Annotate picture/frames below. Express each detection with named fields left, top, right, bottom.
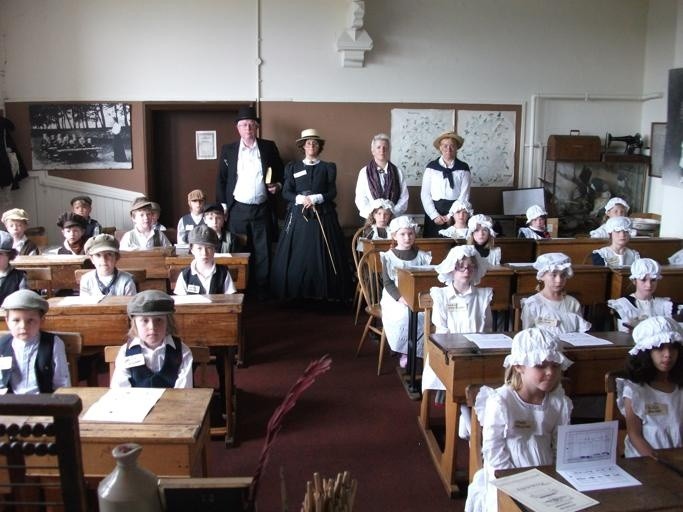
left=648, top=122, right=666, bottom=178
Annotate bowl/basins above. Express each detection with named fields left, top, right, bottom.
left=629, top=217, right=660, bottom=231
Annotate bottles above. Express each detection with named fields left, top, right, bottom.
left=95, top=441, right=164, bottom=512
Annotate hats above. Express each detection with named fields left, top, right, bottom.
left=368, top=198, right=501, bottom=285
left=0, top=207, right=30, bottom=251
left=125, top=289, right=176, bottom=318
left=56, top=195, right=120, bottom=257
left=129, top=190, right=223, bottom=247
left=525, top=205, right=547, bottom=223
left=629, top=317, right=681, bottom=356
left=503, top=326, right=573, bottom=371
left=2, top=289, right=50, bottom=312
left=603, top=197, right=630, bottom=214
left=295, top=128, right=325, bottom=146
left=234, top=108, right=261, bottom=124
left=603, top=214, right=631, bottom=233
left=629, top=258, right=662, bottom=281
left=534, top=253, right=574, bottom=281
left=434, top=132, right=464, bottom=152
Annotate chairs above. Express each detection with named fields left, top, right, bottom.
left=351, top=226, right=367, bottom=327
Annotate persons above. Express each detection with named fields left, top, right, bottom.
left=465, top=214, right=502, bottom=267
left=592, top=216, right=641, bottom=267
left=40, top=132, right=98, bottom=163
left=365, top=199, right=396, bottom=302
left=616, top=316, right=681, bottom=458
left=438, top=199, right=474, bottom=239
left=177, top=192, right=237, bottom=253
left=79, top=233, right=138, bottom=296
left=216, top=106, right=285, bottom=302
left=421, top=245, right=496, bottom=462
left=667, top=249, right=681, bottom=265
left=2, top=231, right=29, bottom=305
left=518, top=205, right=552, bottom=240
left=380, top=216, right=436, bottom=369
left=110, top=290, right=193, bottom=389
left=2, top=289, right=71, bottom=394
left=70, top=196, right=102, bottom=245
left=174, top=224, right=236, bottom=419
left=46, top=212, right=95, bottom=297
left=355, top=132, right=409, bottom=222
left=111, top=116, right=128, bottom=161
left=420, top=130, right=472, bottom=239
left=119, top=197, right=172, bottom=252
left=270, top=129, right=352, bottom=304
left=519, top=253, right=591, bottom=337
left=465, top=328, right=573, bottom=509
left=608, top=258, right=681, bottom=333
left=2, top=208, right=45, bottom=296
left=589, top=199, right=637, bottom=238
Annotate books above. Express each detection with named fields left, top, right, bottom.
left=265, top=166, right=273, bottom=184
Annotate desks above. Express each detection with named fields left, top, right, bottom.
left=41, top=143, right=104, bottom=165
left=359, top=213, right=683, bottom=512
left=0, top=230, right=250, bottom=479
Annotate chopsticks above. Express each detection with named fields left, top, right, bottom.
left=299, top=470, right=358, bottom=511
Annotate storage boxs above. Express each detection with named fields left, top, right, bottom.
left=547, top=133, right=602, bottom=161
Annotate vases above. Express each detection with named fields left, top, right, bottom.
left=97, top=443, right=162, bottom=511
